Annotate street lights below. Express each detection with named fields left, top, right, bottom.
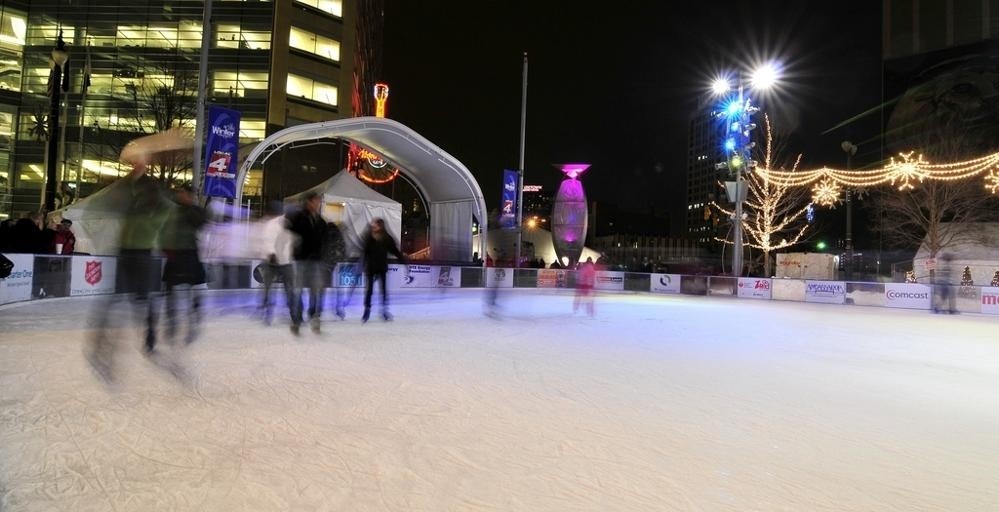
left=714, top=69, right=776, bottom=295
left=841, top=141, right=857, bottom=294
left=32, top=27, right=69, bottom=296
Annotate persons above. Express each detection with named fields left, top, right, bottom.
left=594, top=251, right=609, bottom=270
left=48, top=218, right=76, bottom=255
left=571, top=256, right=596, bottom=319
left=4, top=211, right=48, bottom=253
left=151, top=181, right=207, bottom=347
left=582, top=257, right=594, bottom=270
left=484, top=251, right=492, bottom=267
left=929, top=253, right=963, bottom=316
left=550, top=259, right=561, bottom=269
left=307, top=220, right=348, bottom=319
left=628, top=256, right=669, bottom=273
left=258, top=204, right=307, bottom=324
left=472, top=251, right=484, bottom=266
left=357, top=217, right=406, bottom=322
left=495, top=255, right=503, bottom=267
left=83, top=131, right=173, bottom=375
left=538, top=257, right=546, bottom=268
left=280, top=189, right=332, bottom=334
